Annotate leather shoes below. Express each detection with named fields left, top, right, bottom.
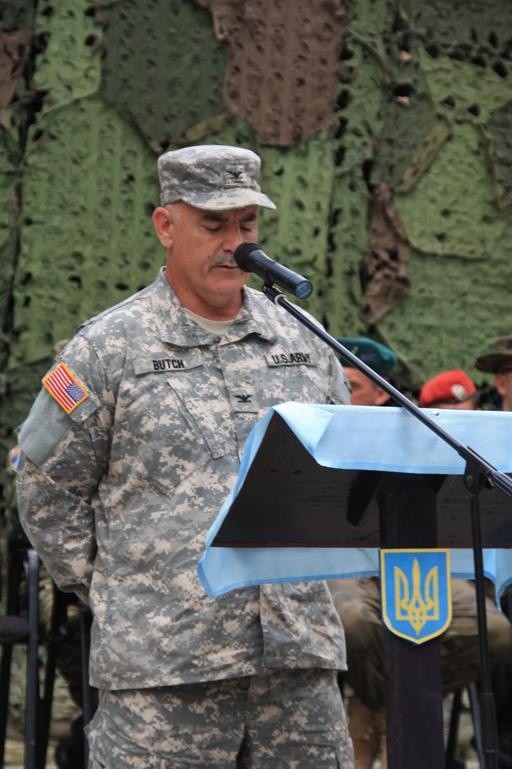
left=53, top=715, right=89, bottom=768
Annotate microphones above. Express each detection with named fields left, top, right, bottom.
left=233, top=241, right=313, bottom=299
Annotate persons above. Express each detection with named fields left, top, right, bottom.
left=17, top=141, right=357, bottom=769
left=1, top=332, right=512, bottom=769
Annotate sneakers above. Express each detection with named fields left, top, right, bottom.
left=346, top=698, right=389, bottom=768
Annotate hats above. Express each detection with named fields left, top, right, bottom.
left=332, top=335, right=398, bottom=376
left=158, top=145, right=277, bottom=213
left=475, top=336, right=512, bottom=374
left=418, top=368, right=477, bottom=408
